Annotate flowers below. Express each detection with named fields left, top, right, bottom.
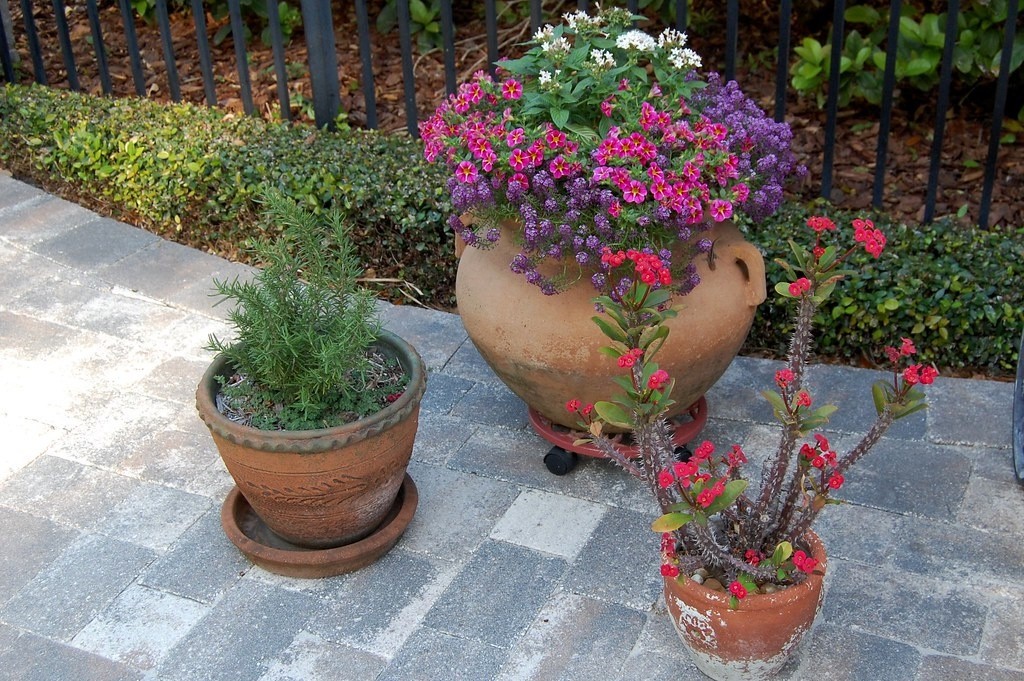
left=567, top=216, right=939, bottom=610
left=416, top=2, right=807, bottom=321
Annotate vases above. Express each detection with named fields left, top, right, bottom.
left=660, top=513, right=827, bottom=681
left=454, top=208, right=767, bottom=437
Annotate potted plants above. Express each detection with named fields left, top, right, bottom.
left=196, top=182, right=427, bottom=548
